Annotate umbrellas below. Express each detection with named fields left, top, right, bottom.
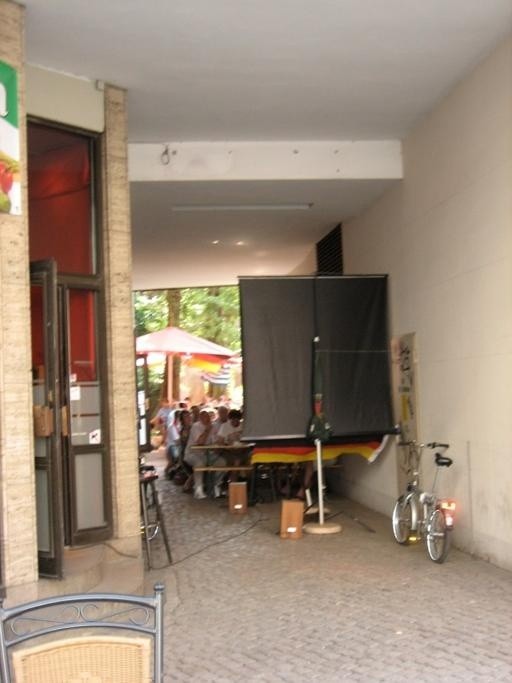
left=137, top=324, right=234, bottom=404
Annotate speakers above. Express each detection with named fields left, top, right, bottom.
left=281, top=499, right=305, bottom=540
left=229, top=482, right=247, bottom=514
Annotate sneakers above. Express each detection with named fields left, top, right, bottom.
left=193, top=480, right=223, bottom=499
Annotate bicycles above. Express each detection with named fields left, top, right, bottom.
left=392, top=440, right=456, bottom=563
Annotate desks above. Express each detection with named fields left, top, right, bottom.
left=191, top=441, right=344, bottom=502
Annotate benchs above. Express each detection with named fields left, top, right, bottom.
left=192, top=465, right=345, bottom=505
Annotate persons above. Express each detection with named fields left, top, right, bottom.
left=279, top=460, right=315, bottom=501
left=148, top=395, right=249, bottom=500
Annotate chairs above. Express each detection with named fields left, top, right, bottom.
left=0, top=583, right=165, bottom=683
